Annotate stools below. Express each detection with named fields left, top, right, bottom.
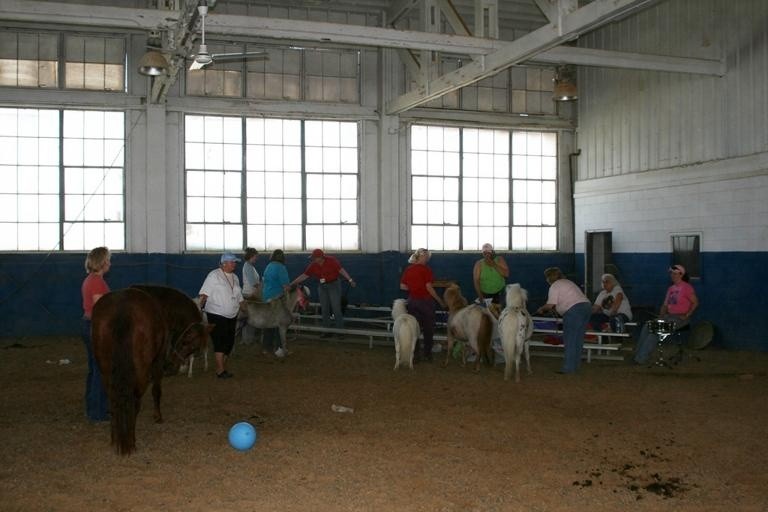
left=668, top=325, right=699, bottom=366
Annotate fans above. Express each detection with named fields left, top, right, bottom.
left=151, top=5, right=274, bottom=73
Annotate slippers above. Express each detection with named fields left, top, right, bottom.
left=217, top=369, right=234, bottom=378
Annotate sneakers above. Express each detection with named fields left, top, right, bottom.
left=319, top=332, right=333, bottom=338
left=336, top=333, right=345, bottom=339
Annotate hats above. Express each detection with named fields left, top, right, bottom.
left=220, top=251, right=242, bottom=265
left=668, top=264, right=686, bottom=275
left=481, top=243, right=495, bottom=254
left=310, top=248, right=324, bottom=256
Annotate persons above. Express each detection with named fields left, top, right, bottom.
left=400, top=248, right=445, bottom=363
left=80, top=246, right=113, bottom=421
left=198, top=253, right=247, bottom=378
left=289, top=249, right=356, bottom=340
left=261, top=249, right=291, bottom=353
left=633, top=264, right=699, bottom=366
left=590, top=274, right=633, bottom=344
left=537, top=267, right=593, bottom=374
left=241, top=248, right=260, bottom=300
left=473, top=243, right=510, bottom=305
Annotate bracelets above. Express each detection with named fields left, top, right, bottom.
left=349, top=278, right=353, bottom=283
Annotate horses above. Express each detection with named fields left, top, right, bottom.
left=182, top=295, right=210, bottom=379
left=442, top=283, right=494, bottom=373
left=498, top=283, right=534, bottom=382
left=89, top=284, right=217, bottom=460
left=236, top=282, right=312, bottom=356
left=389, top=297, right=421, bottom=376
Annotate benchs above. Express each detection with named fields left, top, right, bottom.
left=290, top=299, right=640, bottom=368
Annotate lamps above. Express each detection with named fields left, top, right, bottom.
left=139, top=23, right=173, bottom=79
left=549, top=63, right=582, bottom=105
